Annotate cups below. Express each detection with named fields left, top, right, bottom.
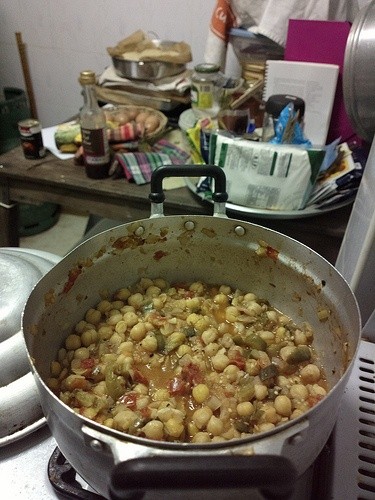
left=217, top=105, right=251, bottom=137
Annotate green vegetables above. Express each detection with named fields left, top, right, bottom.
left=72, top=299, right=313, bottom=434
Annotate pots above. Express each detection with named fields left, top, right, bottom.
left=21, top=164, right=363, bottom=500
left=113, top=56, right=186, bottom=80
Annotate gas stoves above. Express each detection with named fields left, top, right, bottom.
left=48, top=340, right=375, bottom=500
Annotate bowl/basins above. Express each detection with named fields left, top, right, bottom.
left=184, top=144, right=355, bottom=218
left=221, top=74, right=245, bottom=97
left=104, top=105, right=169, bottom=145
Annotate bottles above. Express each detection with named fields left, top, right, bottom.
left=262, top=95, right=305, bottom=143
left=80, top=71, right=115, bottom=180
left=190, top=63, right=225, bottom=120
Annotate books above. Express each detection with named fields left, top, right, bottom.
left=285, top=19, right=362, bottom=149
left=263, top=60, right=339, bottom=150
left=307, top=137, right=364, bottom=209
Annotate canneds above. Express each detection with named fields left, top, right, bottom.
left=18, top=120, right=45, bottom=159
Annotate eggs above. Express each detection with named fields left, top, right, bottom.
left=103, top=107, right=160, bottom=133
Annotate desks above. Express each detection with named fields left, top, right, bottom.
left=0, top=111, right=214, bottom=249
